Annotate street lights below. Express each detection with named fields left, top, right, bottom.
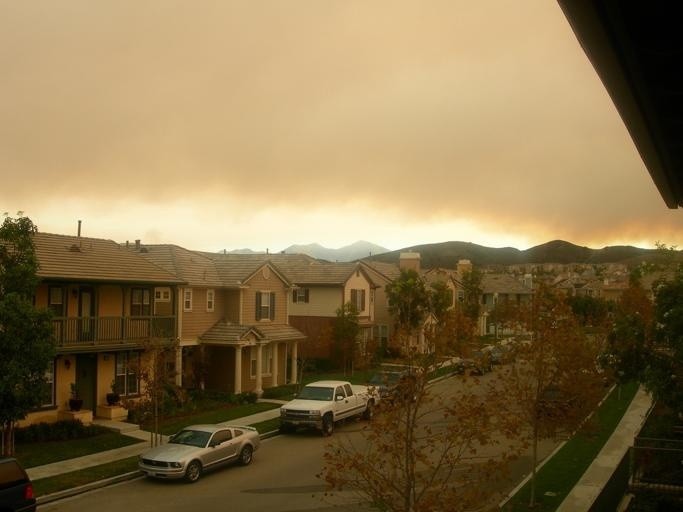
left=153, top=337, right=184, bottom=446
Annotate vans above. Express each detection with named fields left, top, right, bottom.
left=1, top=457, right=36, bottom=512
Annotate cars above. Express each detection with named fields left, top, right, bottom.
left=136, top=423, right=260, bottom=481
left=454, top=337, right=535, bottom=376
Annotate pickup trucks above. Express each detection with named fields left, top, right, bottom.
left=280, top=380, right=379, bottom=437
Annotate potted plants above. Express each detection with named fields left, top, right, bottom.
left=106, top=380, right=124, bottom=403
left=68, top=383, right=82, bottom=411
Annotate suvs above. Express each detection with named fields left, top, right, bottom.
left=369, top=370, right=417, bottom=406
